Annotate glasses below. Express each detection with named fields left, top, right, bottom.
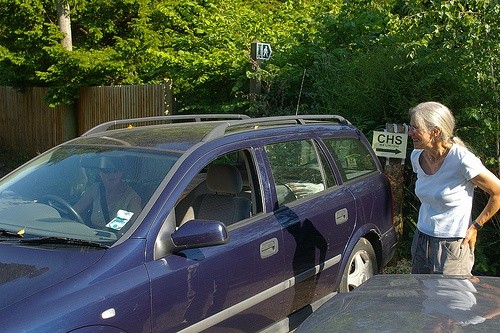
left=408, top=125, right=423, bottom=134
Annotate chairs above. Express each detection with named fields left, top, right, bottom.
left=188, top=163, right=251, bottom=226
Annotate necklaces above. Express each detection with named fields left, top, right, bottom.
left=428, top=146, right=448, bottom=165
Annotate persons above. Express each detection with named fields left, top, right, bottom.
left=69, top=168, right=141, bottom=230
left=416, top=275, right=500, bottom=333
left=410, top=102, right=500, bottom=275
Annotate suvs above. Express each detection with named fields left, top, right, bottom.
left=0, top=115, right=397, bottom=333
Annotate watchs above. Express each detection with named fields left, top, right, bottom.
left=473, top=220, right=484, bottom=231
